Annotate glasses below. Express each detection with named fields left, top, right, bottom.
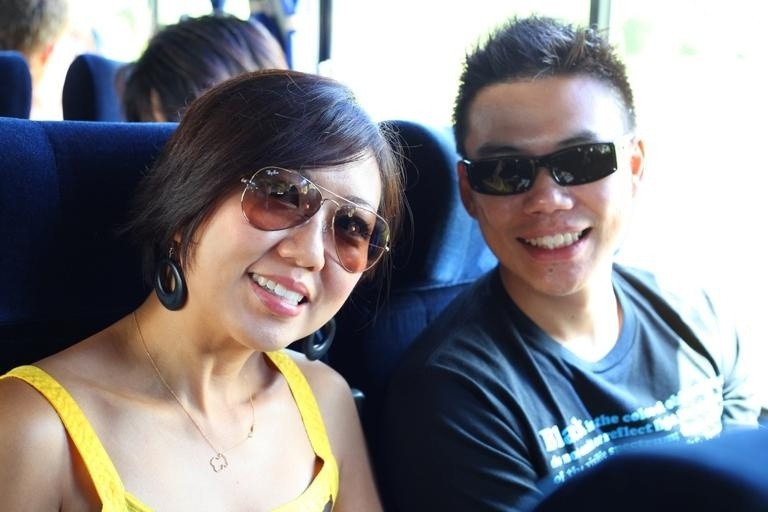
left=460, top=130, right=635, bottom=198
left=239, top=166, right=393, bottom=274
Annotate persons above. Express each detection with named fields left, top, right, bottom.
left=0, top=0, right=74, bottom=124
left=115, top=10, right=289, bottom=121
left=385, top=15, right=763, bottom=509
left=2, top=66, right=409, bottom=511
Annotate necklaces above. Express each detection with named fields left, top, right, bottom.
left=132, top=309, right=259, bottom=473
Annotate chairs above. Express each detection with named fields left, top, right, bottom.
left=0, top=53, right=768, bottom=512
